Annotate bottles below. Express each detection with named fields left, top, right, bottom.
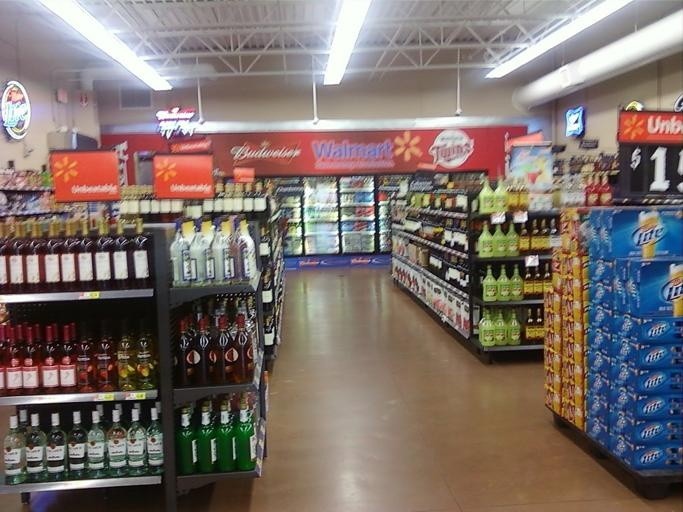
left=477, top=170, right=612, bottom=348
left=0, top=179, right=284, bottom=486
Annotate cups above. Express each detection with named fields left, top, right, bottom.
left=668, top=265, right=682, bottom=317
left=637, top=212, right=657, bottom=259
